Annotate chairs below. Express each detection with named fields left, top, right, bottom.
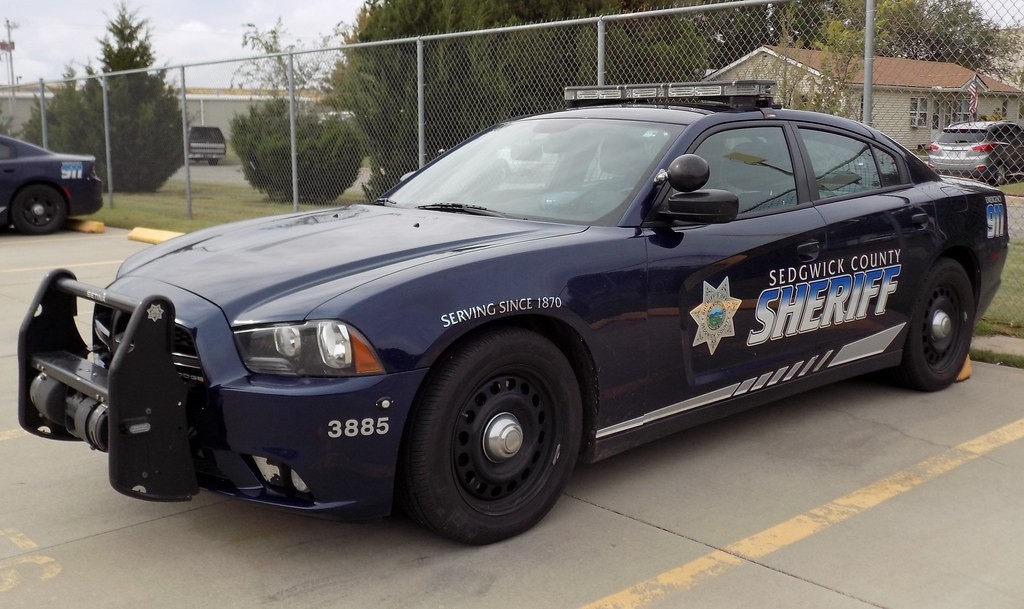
left=725, top=142, right=778, bottom=209
left=598, top=131, right=644, bottom=182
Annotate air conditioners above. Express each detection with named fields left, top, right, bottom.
left=910, top=116, right=925, bottom=128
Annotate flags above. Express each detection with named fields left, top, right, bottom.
left=962, top=75, right=979, bottom=113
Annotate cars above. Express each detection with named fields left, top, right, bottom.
left=187, top=125, right=229, bottom=166
left=927, top=118, right=1024, bottom=186
left=13, top=80, right=1010, bottom=546
left=0, top=135, right=105, bottom=235
